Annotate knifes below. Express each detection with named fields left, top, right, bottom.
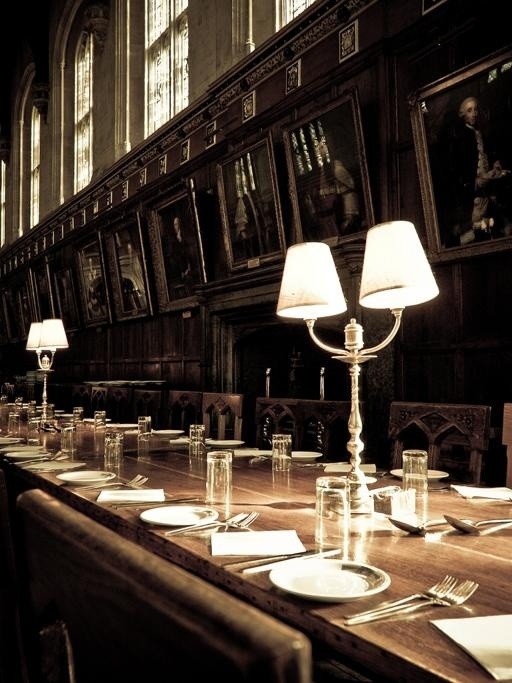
left=112, top=496, right=199, bottom=509
left=223, top=547, right=342, bottom=575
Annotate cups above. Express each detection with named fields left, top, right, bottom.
left=313, top=475, right=350, bottom=544
left=272, top=433, right=293, bottom=471
left=0, top=394, right=206, bottom=465
left=207, top=449, right=234, bottom=505
left=400, top=447, right=428, bottom=499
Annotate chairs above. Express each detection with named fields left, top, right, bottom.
left=0, top=386, right=512, bottom=678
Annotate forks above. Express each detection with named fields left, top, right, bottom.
left=343, top=578, right=481, bottom=630
left=82, top=473, right=149, bottom=489
left=342, top=573, right=460, bottom=619
left=165, top=509, right=261, bottom=536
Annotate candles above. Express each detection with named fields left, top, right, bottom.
left=263, top=367, right=271, bottom=397
left=318, top=366, right=327, bottom=400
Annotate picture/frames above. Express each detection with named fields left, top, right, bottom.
left=1, top=209, right=155, bottom=347
left=281, top=86, right=375, bottom=250
left=213, top=130, right=288, bottom=273
left=141, top=176, right=210, bottom=315
left=407, top=43, right=509, bottom=263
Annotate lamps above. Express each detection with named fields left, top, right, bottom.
left=25, top=319, right=72, bottom=423
left=276, top=218, right=441, bottom=517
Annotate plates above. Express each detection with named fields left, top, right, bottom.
left=207, top=439, right=244, bottom=448
left=257, top=450, right=323, bottom=461
left=391, top=467, right=449, bottom=483
left=56, top=469, right=116, bottom=485
left=272, top=559, right=388, bottom=599
left=141, top=504, right=220, bottom=525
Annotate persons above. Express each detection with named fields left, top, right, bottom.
left=447, top=94, right=501, bottom=240
left=167, top=215, right=198, bottom=282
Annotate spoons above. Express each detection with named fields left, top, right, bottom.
left=442, top=514, right=512, bottom=533
left=387, top=513, right=470, bottom=534
